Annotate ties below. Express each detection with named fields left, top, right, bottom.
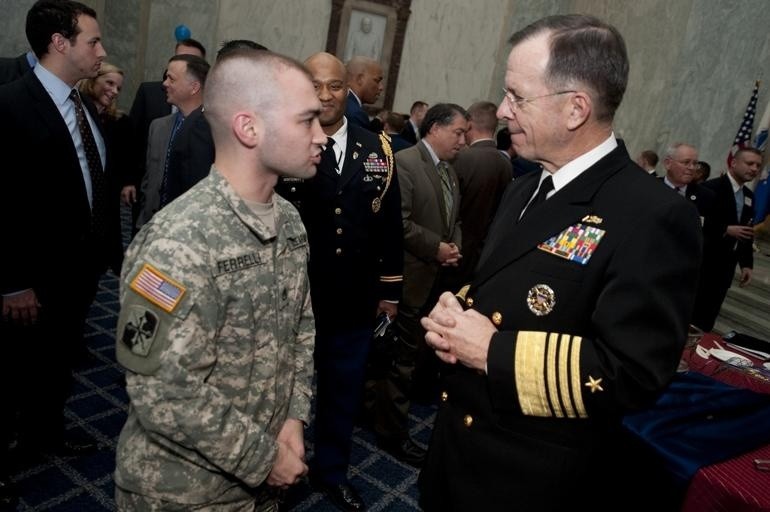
left=439, top=162, right=459, bottom=232
left=519, top=175, right=554, bottom=221
left=323, top=136, right=340, bottom=171
left=69, top=89, right=107, bottom=210
left=160, top=118, right=185, bottom=208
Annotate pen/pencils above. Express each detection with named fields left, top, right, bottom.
left=733, top=217, right=753, bottom=251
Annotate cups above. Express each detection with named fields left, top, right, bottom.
left=675, top=324, right=704, bottom=373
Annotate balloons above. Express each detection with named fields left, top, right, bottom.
left=173, top=25, right=192, bottom=43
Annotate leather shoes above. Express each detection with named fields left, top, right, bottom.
left=376, top=436, right=427, bottom=468
left=1, top=480, right=21, bottom=510
left=35, top=434, right=102, bottom=456
left=309, top=471, right=367, bottom=510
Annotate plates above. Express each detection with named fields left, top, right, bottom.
left=711, top=348, right=754, bottom=370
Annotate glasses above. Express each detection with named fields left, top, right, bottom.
left=502, top=84, right=578, bottom=111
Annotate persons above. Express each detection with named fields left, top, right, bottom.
left=0, top=45, right=39, bottom=85
left=1, top=2, right=122, bottom=474
left=77, top=11, right=768, bottom=510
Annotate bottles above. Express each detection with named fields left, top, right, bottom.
left=742, top=217, right=755, bottom=240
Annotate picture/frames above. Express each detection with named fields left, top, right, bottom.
left=325, top=0, right=412, bottom=116
